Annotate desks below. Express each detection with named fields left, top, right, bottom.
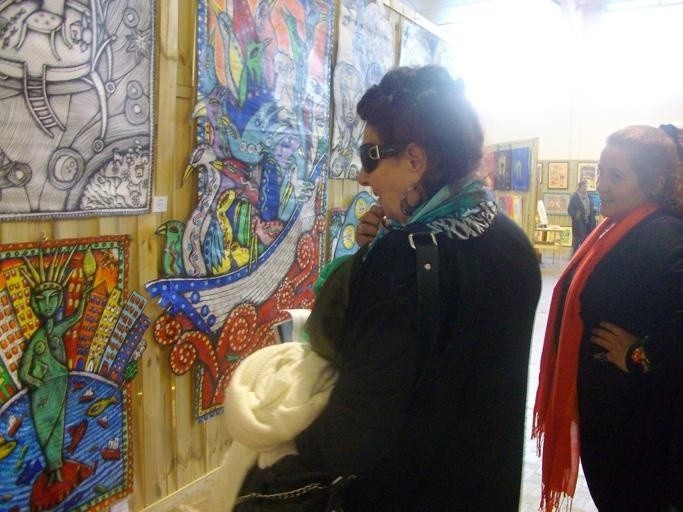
left=535, top=224, right=565, bottom=267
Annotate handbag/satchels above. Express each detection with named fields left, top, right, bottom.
left=232, top=455, right=331, bottom=511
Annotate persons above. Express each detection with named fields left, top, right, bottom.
left=529, top=124, right=682, bottom=512
left=567, top=180, right=597, bottom=253
left=201, top=66, right=540, bottom=512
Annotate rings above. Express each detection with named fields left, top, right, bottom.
left=599, top=352, right=607, bottom=363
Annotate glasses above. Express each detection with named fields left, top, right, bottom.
left=358, top=138, right=405, bottom=174
